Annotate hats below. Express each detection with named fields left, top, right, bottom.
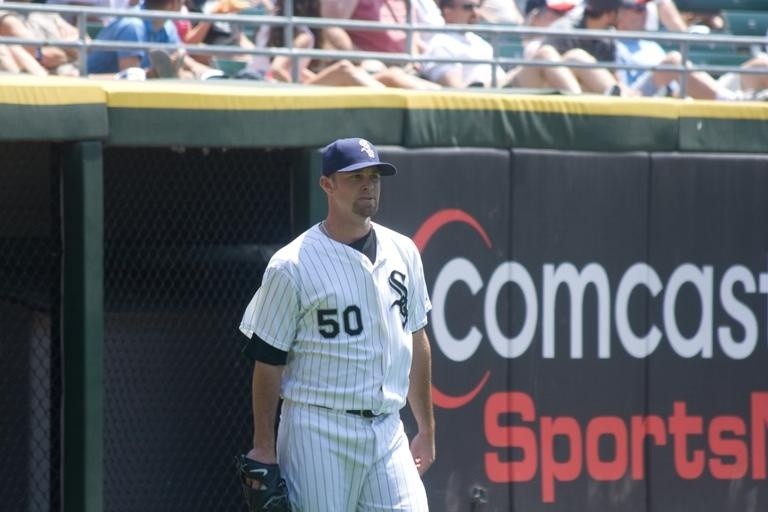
left=317, top=134, right=400, bottom=180
left=543, top=0, right=578, bottom=13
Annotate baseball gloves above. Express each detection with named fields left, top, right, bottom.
left=236, top=453, right=292, bottom=512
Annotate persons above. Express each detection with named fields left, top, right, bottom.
left=235, top=138, right=437, bottom=512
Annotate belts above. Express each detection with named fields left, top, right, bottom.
left=303, top=399, right=386, bottom=420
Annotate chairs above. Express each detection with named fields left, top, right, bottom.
left=79, top=0, right=767, bottom=105
